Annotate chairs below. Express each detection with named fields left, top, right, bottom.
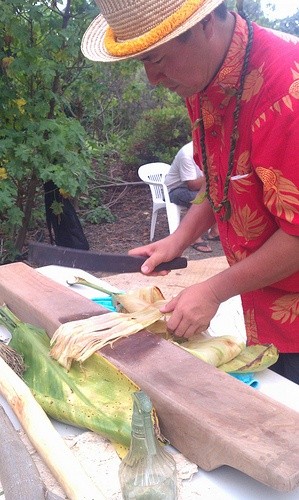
left=138, top=162, right=187, bottom=241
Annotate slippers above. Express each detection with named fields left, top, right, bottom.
left=190, top=241, right=213, bottom=253
left=208, top=234, right=221, bottom=241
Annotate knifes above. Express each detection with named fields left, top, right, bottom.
left=27, top=241, right=188, bottom=274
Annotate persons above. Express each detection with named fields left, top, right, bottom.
left=81, top=0, right=299, bottom=386
left=161, top=142, right=220, bottom=252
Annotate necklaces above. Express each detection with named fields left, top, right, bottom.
left=199, top=9, right=253, bottom=221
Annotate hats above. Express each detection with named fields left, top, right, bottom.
left=79, top=0, right=231, bottom=63
